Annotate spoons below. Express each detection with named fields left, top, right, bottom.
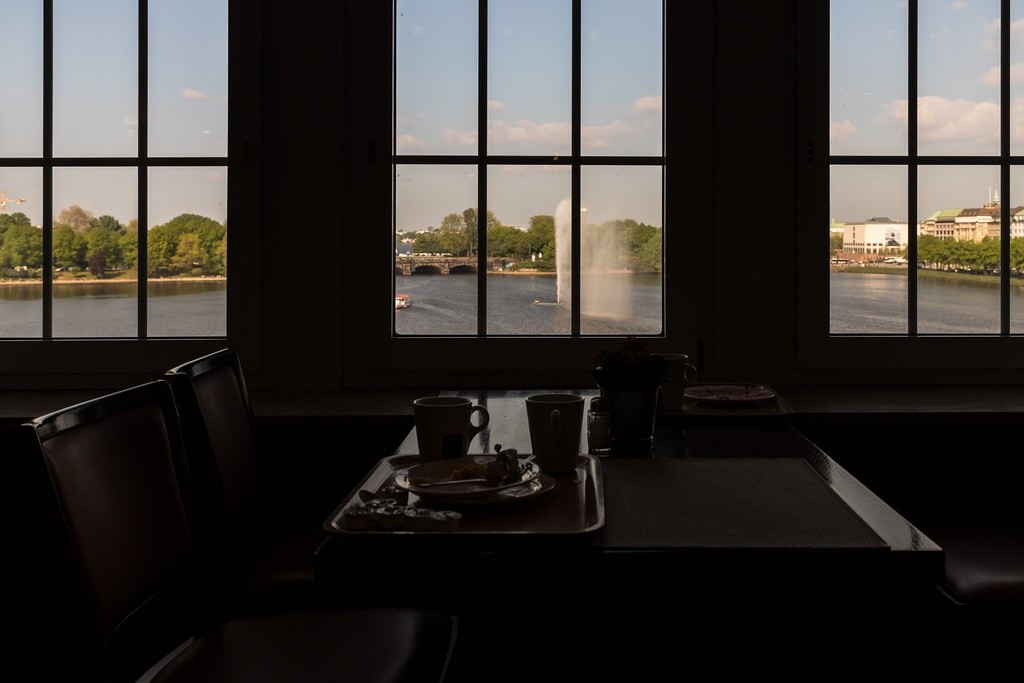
left=416, top=478, right=487, bottom=488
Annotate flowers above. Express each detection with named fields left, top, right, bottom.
left=591, top=339, right=655, bottom=371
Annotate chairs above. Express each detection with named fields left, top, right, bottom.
left=925, top=514, right=1023, bottom=683
left=15, top=379, right=468, bottom=682
left=164, top=348, right=508, bottom=628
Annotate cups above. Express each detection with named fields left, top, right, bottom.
left=526, top=392, right=585, bottom=472
left=650, top=353, right=696, bottom=412
left=412, top=395, right=489, bottom=463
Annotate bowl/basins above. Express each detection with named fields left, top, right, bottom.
left=343, top=486, right=462, bottom=532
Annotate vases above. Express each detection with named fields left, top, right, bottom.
left=590, top=371, right=672, bottom=460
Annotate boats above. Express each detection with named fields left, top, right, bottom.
left=394, top=293, right=410, bottom=310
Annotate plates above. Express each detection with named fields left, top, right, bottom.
left=457, top=473, right=557, bottom=504
left=684, top=385, right=775, bottom=408
left=394, top=456, right=540, bottom=498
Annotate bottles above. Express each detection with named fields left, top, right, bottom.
left=588, top=397, right=613, bottom=458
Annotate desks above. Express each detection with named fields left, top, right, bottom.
left=310, top=387, right=947, bottom=683
